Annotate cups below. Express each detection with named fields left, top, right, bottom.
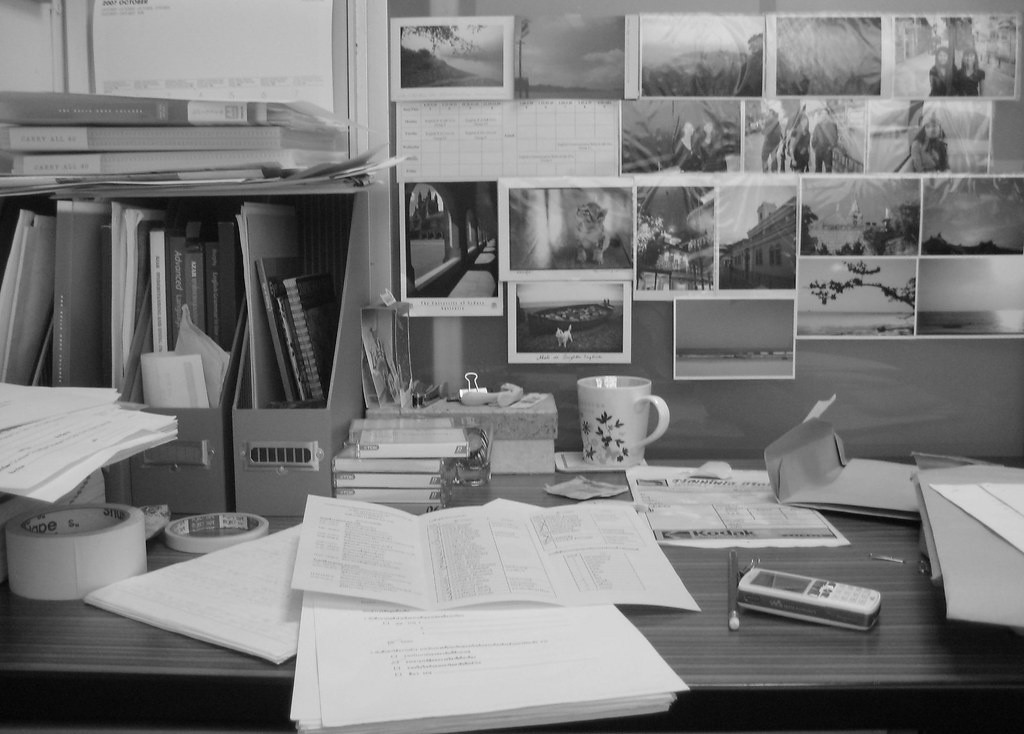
left=575, top=375, right=671, bottom=468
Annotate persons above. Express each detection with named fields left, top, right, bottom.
left=677, top=34, right=984, bottom=170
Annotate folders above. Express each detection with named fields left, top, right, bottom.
left=0, top=87, right=355, bottom=175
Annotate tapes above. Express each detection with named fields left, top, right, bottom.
left=5, top=502, right=148, bottom=601
left=164, top=512, right=270, bottom=553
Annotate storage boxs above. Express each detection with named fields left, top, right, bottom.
left=365, top=391, right=557, bottom=474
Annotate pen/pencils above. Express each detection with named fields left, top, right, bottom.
left=727, top=547, right=740, bottom=631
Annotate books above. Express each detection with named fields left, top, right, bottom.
left=0, top=200, right=1024, bottom=734
left=1, top=91, right=412, bottom=196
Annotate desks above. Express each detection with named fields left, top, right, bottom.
left=0, top=460, right=1024, bottom=689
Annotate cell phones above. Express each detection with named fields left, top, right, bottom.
left=737, top=567, right=882, bottom=631
